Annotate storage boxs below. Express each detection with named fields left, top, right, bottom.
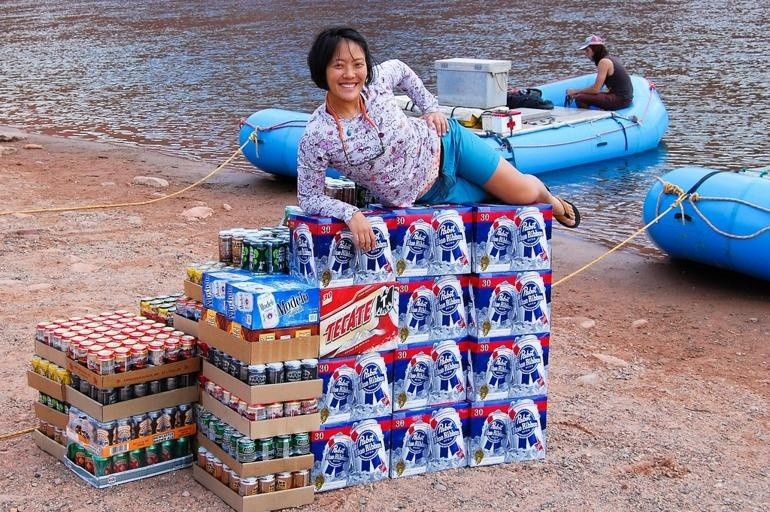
left=435, top=58, right=512, bottom=109
left=202, top=202, right=553, bottom=493
left=481, top=111, right=521, bottom=134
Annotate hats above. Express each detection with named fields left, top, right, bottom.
left=579, top=35, right=604, bottom=50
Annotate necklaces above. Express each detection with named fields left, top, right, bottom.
left=328, top=102, right=361, bottom=137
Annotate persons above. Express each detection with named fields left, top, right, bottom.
left=563, top=33, right=635, bottom=111
left=295, top=26, right=581, bottom=253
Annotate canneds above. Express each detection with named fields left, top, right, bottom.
left=196, top=446, right=309, bottom=496
left=217, top=226, right=291, bottom=275
left=483, top=207, right=553, bottom=271
left=176, top=295, right=202, bottom=322
left=207, top=345, right=318, bottom=386
left=484, top=271, right=550, bottom=327
left=325, top=353, right=388, bottom=413
left=210, top=280, right=227, bottom=299
left=323, top=173, right=375, bottom=210
left=479, top=398, right=544, bottom=454
left=403, top=340, right=463, bottom=401
left=70, top=372, right=194, bottom=404
left=193, top=401, right=309, bottom=463
left=204, top=381, right=318, bottom=421
left=486, top=333, right=545, bottom=391
left=327, top=216, right=395, bottom=281
left=35, top=310, right=195, bottom=375
left=195, top=338, right=209, bottom=390
left=68, top=437, right=194, bottom=477
left=399, top=210, right=472, bottom=275
left=38, top=391, right=70, bottom=416
left=68, top=403, right=193, bottom=447
left=402, top=407, right=466, bottom=463
left=140, top=293, right=186, bottom=327
left=32, top=354, right=67, bottom=386
left=186, top=260, right=234, bottom=286
left=234, top=292, right=254, bottom=312
left=257, top=292, right=279, bottom=329
left=291, top=223, right=316, bottom=280
left=320, top=418, right=387, bottom=480
left=405, top=275, right=467, bottom=334
left=39, top=420, right=68, bottom=447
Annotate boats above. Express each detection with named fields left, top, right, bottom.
left=643, top=165, right=769, bottom=281
left=239, top=66, right=670, bottom=184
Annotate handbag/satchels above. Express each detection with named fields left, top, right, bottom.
left=507, top=89, right=541, bottom=109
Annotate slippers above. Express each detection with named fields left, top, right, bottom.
left=553, top=196, right=580, bottom=229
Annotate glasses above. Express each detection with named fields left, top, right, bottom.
left=340, top=116, right=385, bottom=167
